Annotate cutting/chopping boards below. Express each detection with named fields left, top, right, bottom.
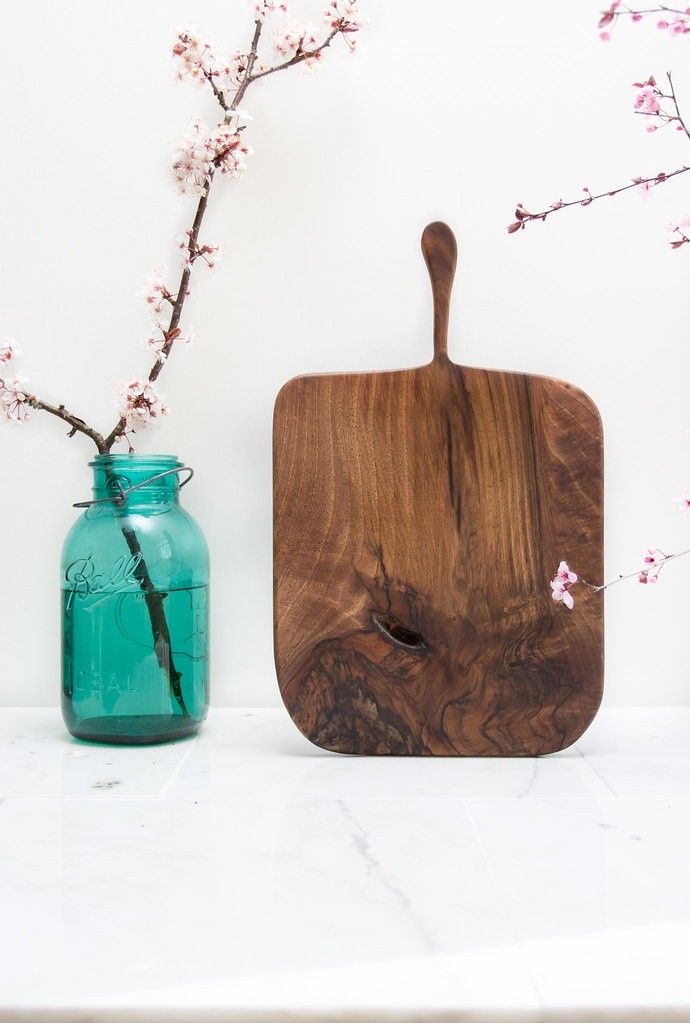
left=273, top=220, right=606, bottom=757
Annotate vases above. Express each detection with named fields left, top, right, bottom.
left=59, top=455, right=209, bottom=739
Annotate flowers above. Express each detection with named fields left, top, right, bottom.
left=505, top=1, right=690, bottom=264
left=0, top=2, right=358, bottom=726
left=550, top=496, right=690, bottom=610
